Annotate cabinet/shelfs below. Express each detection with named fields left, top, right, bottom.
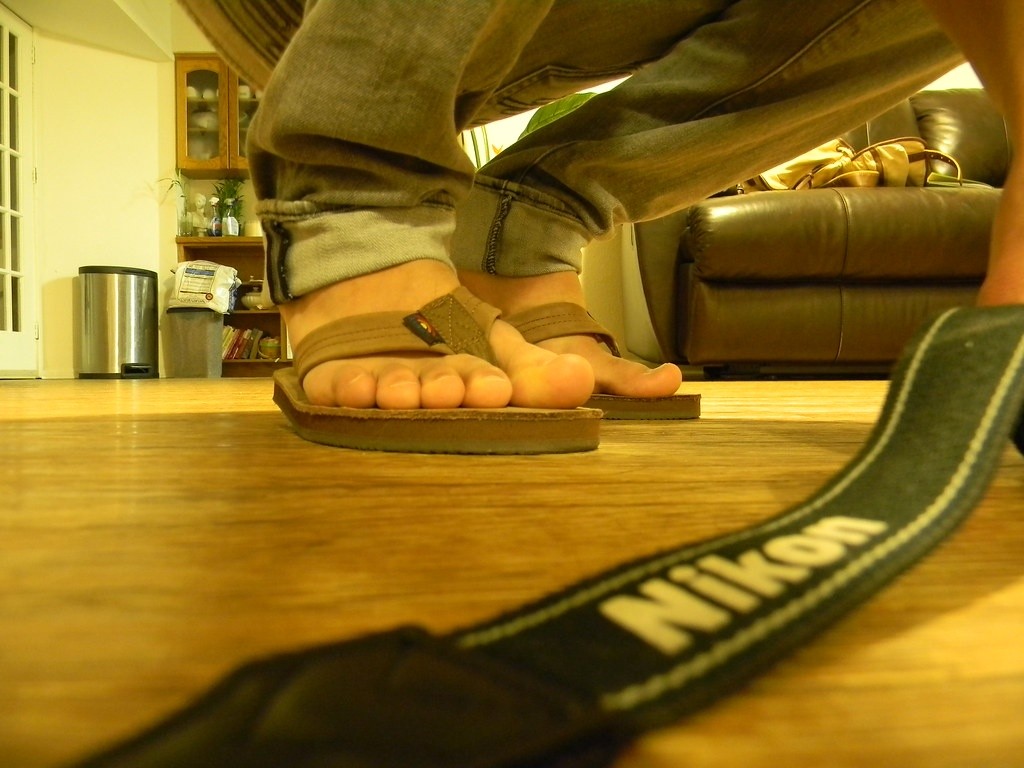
left=174, top=236, right=294, bottom=378
left=174, top=51, right=264, bottom=181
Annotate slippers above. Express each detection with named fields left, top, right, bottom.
left=271, top=281, right=605, bottom=456
left=497, top=301, right=702, bottom=421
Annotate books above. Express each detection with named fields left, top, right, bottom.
left=222, top=325, right=262, bottom=359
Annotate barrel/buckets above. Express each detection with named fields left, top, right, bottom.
left=258, top=343, right=281, bottom=359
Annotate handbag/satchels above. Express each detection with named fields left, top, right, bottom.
left=725, top=136, right=964, bottom=195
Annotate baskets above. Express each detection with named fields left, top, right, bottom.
left=259, top=343, right=282, bottom=359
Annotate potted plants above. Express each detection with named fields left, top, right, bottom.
left=157, top=167, right=195, bottom=236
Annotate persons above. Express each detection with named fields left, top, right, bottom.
left=187, top=193, right=208, bottom=230
left=181, top=0, right=1024, bottom=454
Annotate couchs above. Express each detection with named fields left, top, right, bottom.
left=619, top=89, right=1013, bottom=382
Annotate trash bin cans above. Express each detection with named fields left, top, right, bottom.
left=165, top=306, right=225, bottom=378
left=77, top=264, right=162, bottom=379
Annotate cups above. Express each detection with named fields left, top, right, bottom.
left=239, top=86, right=250, bottom=98
